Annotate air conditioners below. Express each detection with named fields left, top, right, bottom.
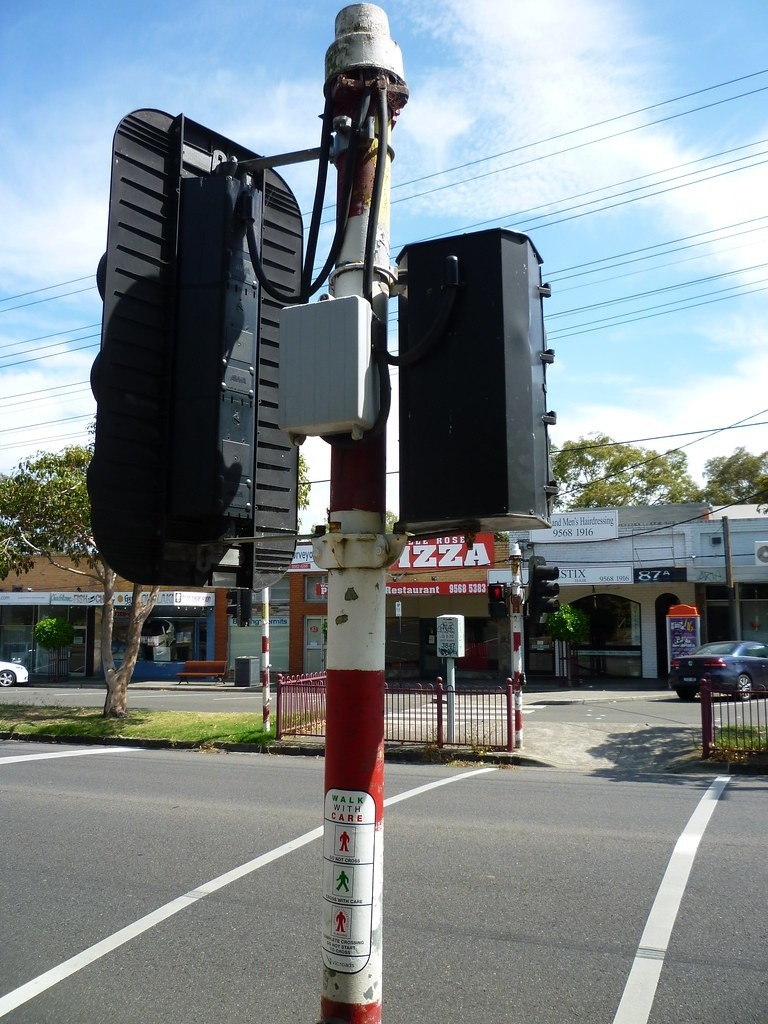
left=755, top=541, right=768, bottom=566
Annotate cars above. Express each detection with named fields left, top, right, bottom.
left=669, top=641, right=768, bottom=702
left=5, top=642, right=35, bottom=670
left=0, top=661, right=29, bottom=686
left=139, top=619, right=176, bottom=647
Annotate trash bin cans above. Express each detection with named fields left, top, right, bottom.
left=235, top=656, right=261, bottom=687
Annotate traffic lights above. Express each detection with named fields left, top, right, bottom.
left=487, top=581, right=508, bottom=618
left=529, top=557, right=560, bottom=623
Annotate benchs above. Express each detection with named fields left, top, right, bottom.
left=176, top=661, right=229, bottom=685
